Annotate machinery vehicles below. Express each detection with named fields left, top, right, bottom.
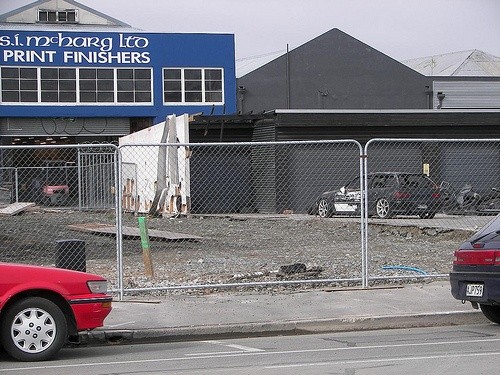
left=37, top=160, right=70, bottom=205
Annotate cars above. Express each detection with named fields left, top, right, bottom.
left=449, top=213, right=500, bottom=324
left=316, top=169, right=444, bottom=218
left=0, top=262, right=114, bottom=361
